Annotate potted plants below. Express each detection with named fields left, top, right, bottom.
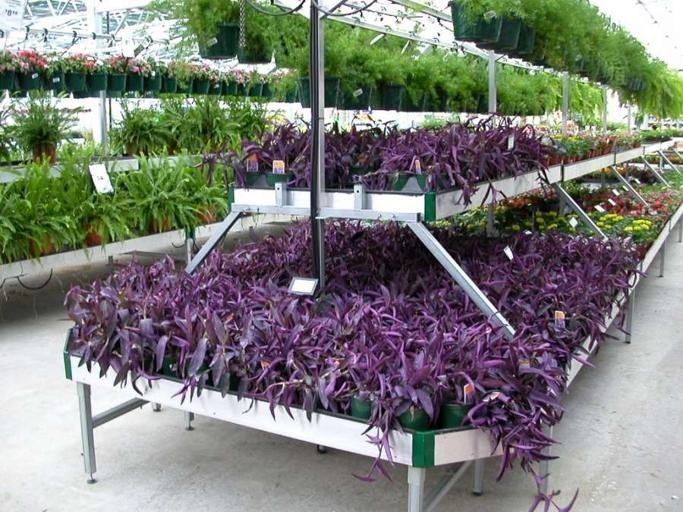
left=0, top=0, right=683, bottom=512
